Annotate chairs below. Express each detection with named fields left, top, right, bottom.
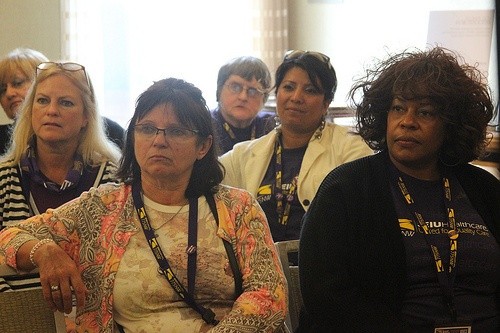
left=0, top=239, right=301, bottom=333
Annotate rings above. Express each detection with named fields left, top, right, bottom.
left=50, top=285, right=59, bottom=291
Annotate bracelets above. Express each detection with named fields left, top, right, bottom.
left=29, top=239, right=57, bottom=267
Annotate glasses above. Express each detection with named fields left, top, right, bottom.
left=132, top=124, right=198, bottom=139
left=284, top=50, right=336, bottom=72
left=35, top=61, right=91, bottom=89
left=224, top=82, right=264, bottom=97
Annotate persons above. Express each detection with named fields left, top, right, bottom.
left=218, top=49, right=382, bottom=267
left=0, top=78, right=291, bottom=333
left=0, top=48, right=126, bottom=232
left=209, top=57, right=281, bottom=157
left=298, top=46, right=500, bottom=333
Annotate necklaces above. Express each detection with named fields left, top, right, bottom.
left=141, top=189, right=188, bottom=231
